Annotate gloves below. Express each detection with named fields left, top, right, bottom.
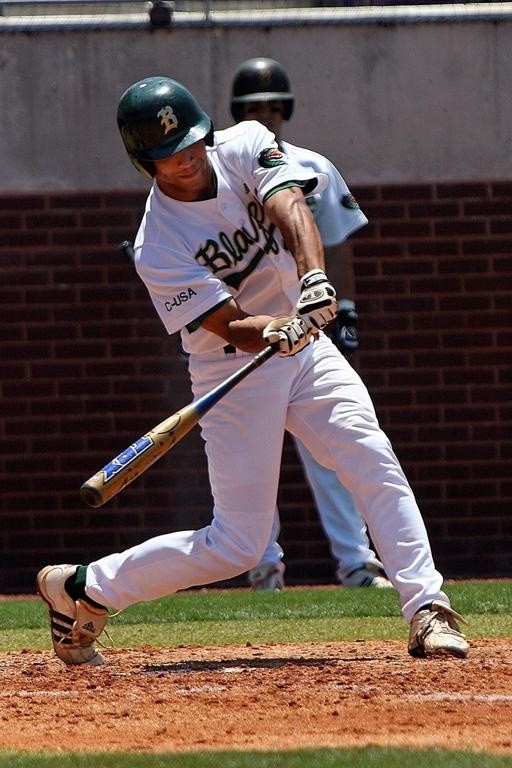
left=328, top=299, right=360, bottom=355
left=262, top=316, right=318, bottom=357
left=295, top=268, right=338, bottom=331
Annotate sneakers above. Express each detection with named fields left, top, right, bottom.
left=408, top=599, right=470, bottom=657
left=342, top=561, right=393, bottom=589
left=249, top=562, right=286, bottom=595
left=37, top=562, right=114, bottom=667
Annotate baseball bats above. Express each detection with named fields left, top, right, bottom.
left=79, top=341, right=280, bottom=508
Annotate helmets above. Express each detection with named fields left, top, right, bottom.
left=116, top=77, right=214, bottom=180
left=229, top=56, right=296, bottom=123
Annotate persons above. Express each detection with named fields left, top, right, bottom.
left=32, top=75, right=472, bottom=666
left=224, top=51, right=402, bottom=590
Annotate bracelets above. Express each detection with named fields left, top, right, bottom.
left=333, top=298, right=356, bottom=312
